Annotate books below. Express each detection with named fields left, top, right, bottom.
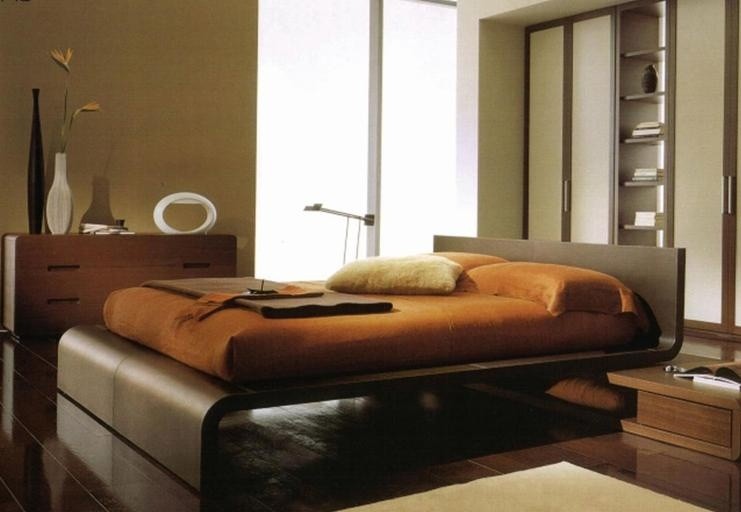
left=671, top=353, right=741, bottom=394
left=79, top=223, right=135, bottom=236
left=627, top=118, right=667, bottom=230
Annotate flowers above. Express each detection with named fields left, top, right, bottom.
left=48, top=47, right=100, bottom=152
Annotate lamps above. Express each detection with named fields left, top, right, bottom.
left=302, top=202, right=375, bottom=265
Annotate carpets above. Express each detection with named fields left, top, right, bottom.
left=334, top=461, right=715, bottom=512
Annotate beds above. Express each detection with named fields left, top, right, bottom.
left=57, top=235, right=685, bottom=491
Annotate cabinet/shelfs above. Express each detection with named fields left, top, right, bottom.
left=524, top=0, right=741, bottom=344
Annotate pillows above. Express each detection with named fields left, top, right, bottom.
left=457, top=262, right=652, bottom=335
left=325, top=253, right=465, bottom=295
left=430, top=251, right=510, bottom=274
left=547, top=378, right=635, bottom=416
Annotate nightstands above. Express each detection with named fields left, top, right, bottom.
left=607, top=353, right=741, bottom=462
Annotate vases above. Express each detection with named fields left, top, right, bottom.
left=46, top=153, right=73, bottom=235
left=28, top=88, right=44, bottom=235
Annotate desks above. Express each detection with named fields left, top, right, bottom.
left=0, top=234, right=237, bottom=340
